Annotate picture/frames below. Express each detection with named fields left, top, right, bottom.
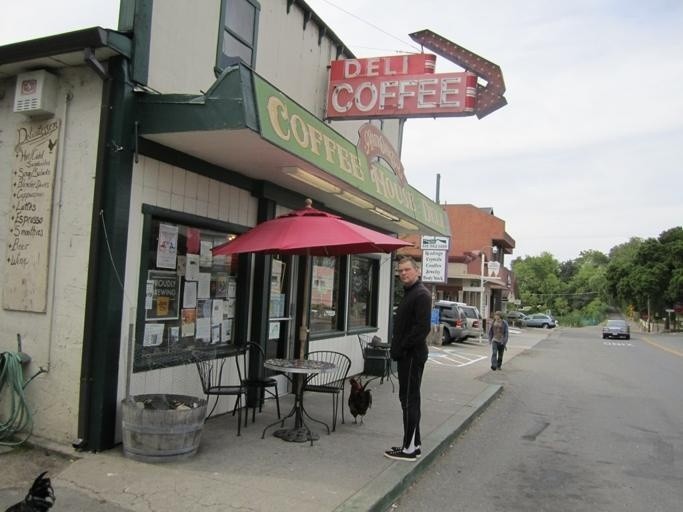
left=145, top=270, right=182, bottom=321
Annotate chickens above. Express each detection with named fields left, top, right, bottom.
left=4, top=470, right=56, bottom=512
left=348, top=377, right=373, bottom=425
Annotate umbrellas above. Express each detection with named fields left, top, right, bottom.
left=210, top=198, right=416, bottom=430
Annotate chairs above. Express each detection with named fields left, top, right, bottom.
left=356, top=332, right=389, bottom=381
left=233, top=342, right=281, bottom=427
left=301, top=351, right=352, bottom=432
left=192, top=349, right=249, bottom=436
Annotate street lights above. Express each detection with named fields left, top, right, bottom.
left=479, top=243, right=499, bottom=331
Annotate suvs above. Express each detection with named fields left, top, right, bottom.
left=437, top=299, right=483, bottom=343
left=516, top=312, right=556, bottom=329
left=435, top=302, right=469, bottom=346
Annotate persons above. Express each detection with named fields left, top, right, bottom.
left=383, top=256, right=432, bottom=462
left=488, top=311, right=509, bottom=371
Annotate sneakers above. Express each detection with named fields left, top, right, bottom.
left=389, top=446, right=421, bottom=457
left=384, top=449, right=416, bottom=461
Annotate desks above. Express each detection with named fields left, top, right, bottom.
left=261, top=359, right=339, bottom=446
left=364, top=343, right=399, bottom=393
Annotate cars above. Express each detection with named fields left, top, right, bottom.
left=507, top=310, right=529, bottom=320
left=600, top=318, right=631, bottom=339
